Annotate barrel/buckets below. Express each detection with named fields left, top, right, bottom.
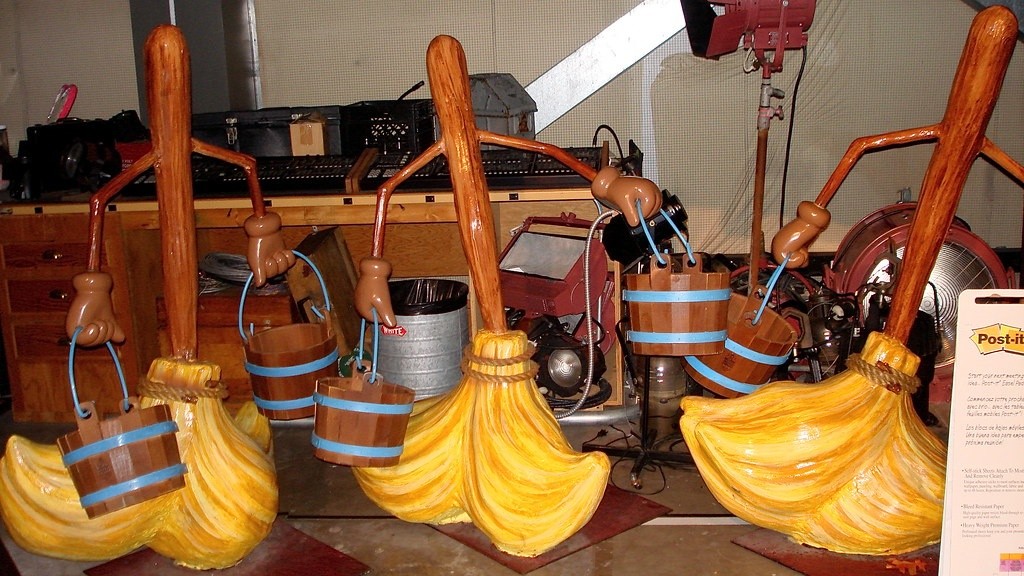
left=623, top=199, right=732, bottom=357
left=238, top=250, right=339, bottom=420
left=55, top=325, right=189, bottom=520
left=310, top=308, right=415, bottom=468
left=679, top=252, right=799, bottom=399
